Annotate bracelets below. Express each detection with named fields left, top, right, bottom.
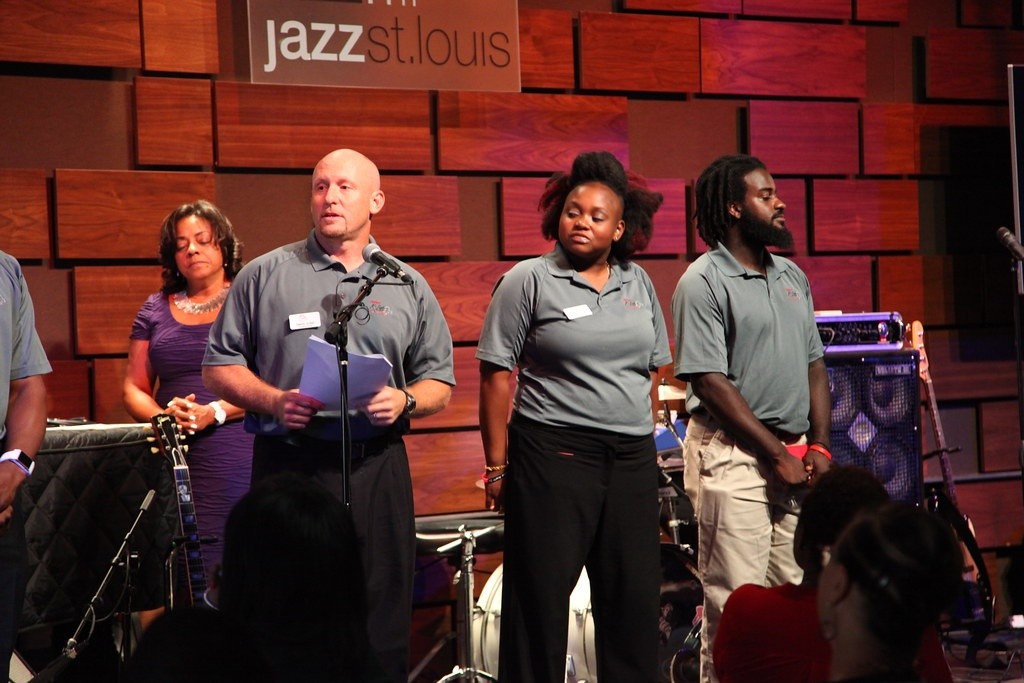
left=810, top=444, right=832, bottom=461
left=484, top=462, right=508, bottom=471
left=488, top=473, right=505, bottom=482
left=813, top=441, right=831, bottom=452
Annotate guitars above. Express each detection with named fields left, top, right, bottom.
left=148, top=413, right=218, bottom=615
left=907, top=319, right=999, bottom=632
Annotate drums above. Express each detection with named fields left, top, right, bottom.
left=464, top=547, right=601, bottom=683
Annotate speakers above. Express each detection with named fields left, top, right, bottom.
left=820, top=349, right=926, bottom=513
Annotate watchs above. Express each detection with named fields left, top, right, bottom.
left=0, top=448, right=36, bottom=477
left=401, top=388, right=417, bottom=416
left=208, top=400, right=227, bottom=427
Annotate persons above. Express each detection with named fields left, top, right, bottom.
left=198, top=149, right=455, bottom=682
left=473, top=152, right=675, bottom=683
left=709, top=468, right=970, bottom=683
left=125, top=468, right=392, bottom=682
left=670, top=155, right=833, bottom=682
left=122, top=199, right=258, bottom=632
left=0, top=248, right=52, bottom=683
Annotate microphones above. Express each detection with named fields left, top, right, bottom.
left=27, top=641, right=86, bottom=683
left=997, top=227, right=1024, bottom=260
left=362, top=243, right=414, bottom=285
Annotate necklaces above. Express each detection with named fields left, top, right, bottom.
left=173, top=278, right=233, bottom=314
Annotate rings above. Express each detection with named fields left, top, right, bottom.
left=371, top=412, right=376, bottom=419
